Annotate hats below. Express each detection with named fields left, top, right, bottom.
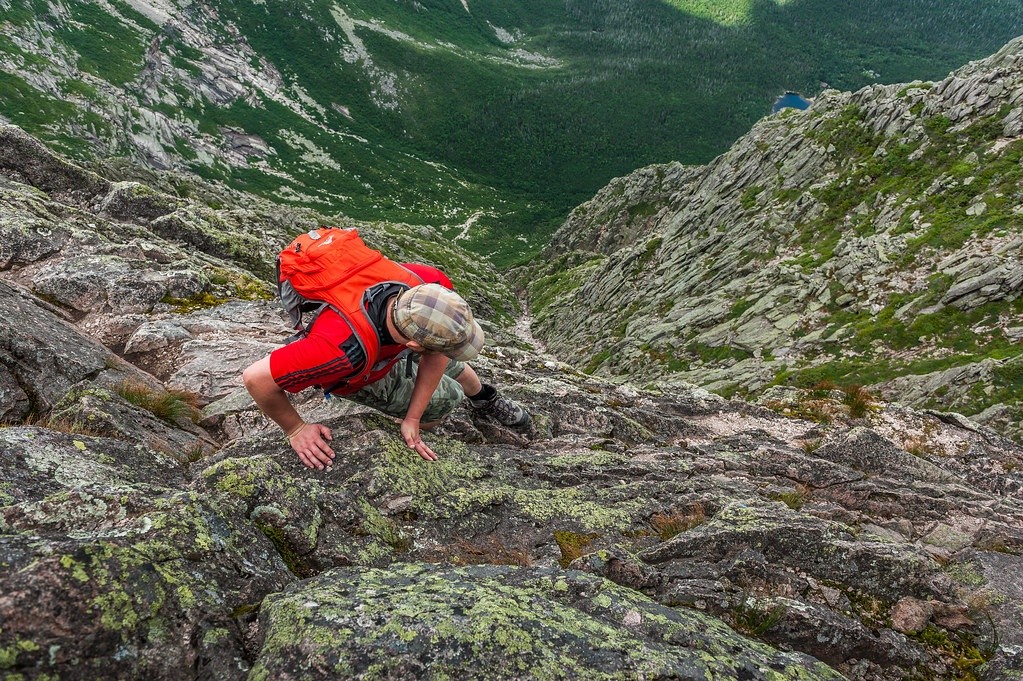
left=394, top=282, right=485, bottom=360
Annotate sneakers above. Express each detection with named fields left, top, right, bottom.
left=466, top=384, right=529, bottom=428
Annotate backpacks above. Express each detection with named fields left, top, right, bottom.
left=276, top=226, right=425, bottom=388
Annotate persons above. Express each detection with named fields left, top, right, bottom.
left=242, top=229, right=531, bottom=471
